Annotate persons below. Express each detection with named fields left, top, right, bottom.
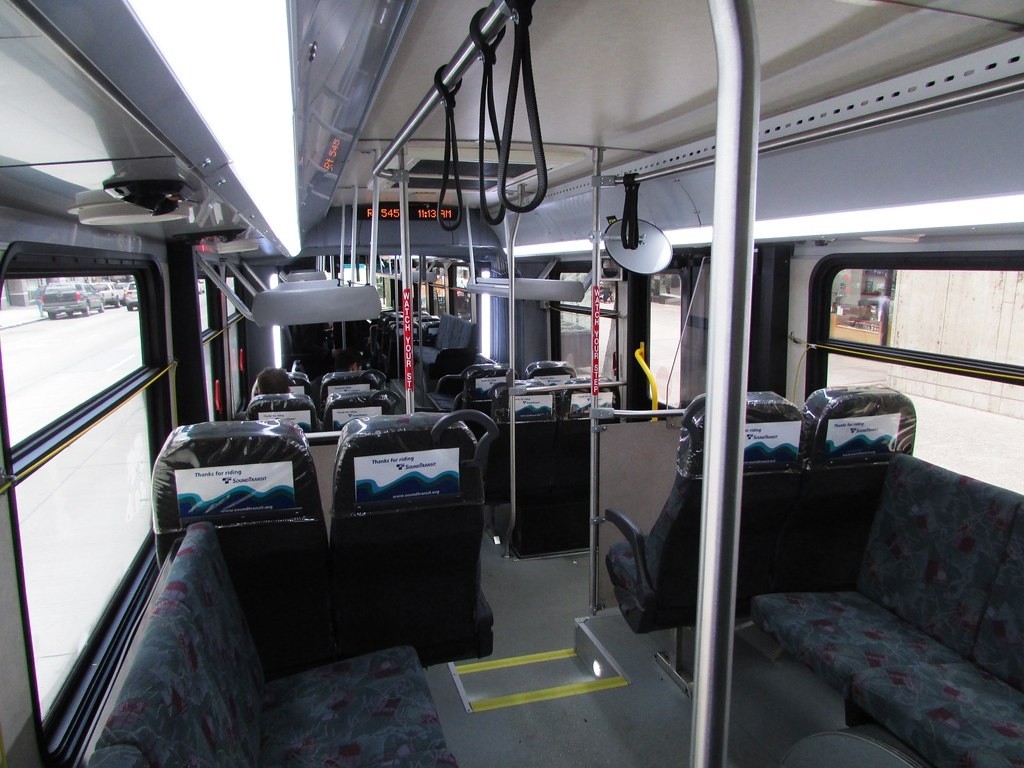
left=333, top=349, right=366, bottom=371
left=254, top=368, right=292, bottom=395
left=34, top=285, right=44, bottom=317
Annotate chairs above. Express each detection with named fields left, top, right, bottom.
left=86, top=301, right=1024, bottom=764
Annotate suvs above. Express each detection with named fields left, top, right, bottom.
left=92, top=282, right=126, bottom=309
left=42, top=282, right=105, bottom=320
left=123, top=280, right=139, bottom=312
